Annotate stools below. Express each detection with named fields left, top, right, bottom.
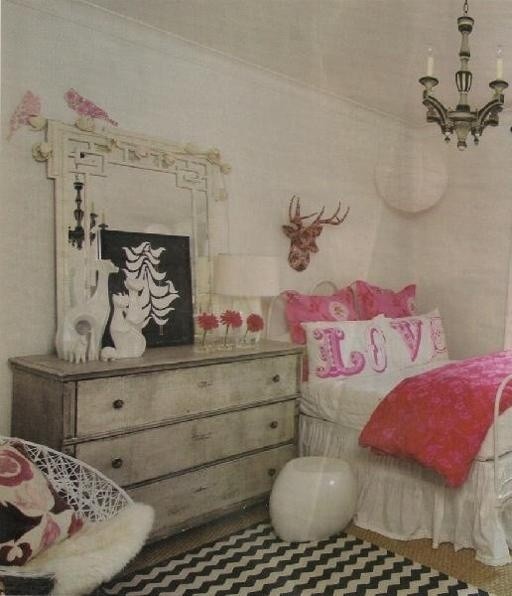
left=267, top=455, right=357, bottom=544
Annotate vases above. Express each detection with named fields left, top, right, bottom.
left=214, top=329, right=236, bottom=353
left=189, top=332, right=216, bottom=355
left=234, top=326, right=263, bottom=350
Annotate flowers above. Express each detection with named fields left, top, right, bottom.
left=196, top=311, right=219, bottom=337
left=219, top=308, right=244, bottom=335
left=242, top=311, right=264, bottom=336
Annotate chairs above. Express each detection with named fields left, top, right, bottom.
left=1, top=433, right=157, bottom=595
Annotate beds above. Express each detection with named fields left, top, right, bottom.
left=261, top=275, right=512, bottom=571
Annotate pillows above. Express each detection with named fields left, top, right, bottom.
left=294, top=313, right=391, bottom=390
left=351, top=277, right=420, bottom=320
left=275, top=283, right=362, bottom=388
left=0, top=438, right=86, bottom=571
left=368, top=305, right=449, bottom=373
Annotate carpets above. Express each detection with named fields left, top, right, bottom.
left=88, top=517, right=496, bottom=595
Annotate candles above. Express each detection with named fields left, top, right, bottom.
left=90, top=198, right=96, bottom=214
left=99, top=209, right=107, bottom=225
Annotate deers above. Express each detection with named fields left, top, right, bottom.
left=282, top=195, right=351, bottom=272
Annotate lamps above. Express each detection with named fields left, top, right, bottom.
left=417, top=0, right=510, bottom=153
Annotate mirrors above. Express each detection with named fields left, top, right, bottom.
left=31, top=111, right=233, bottom=365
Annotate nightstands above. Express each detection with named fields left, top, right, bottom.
left=5, top=333, right=316, bottom=588
